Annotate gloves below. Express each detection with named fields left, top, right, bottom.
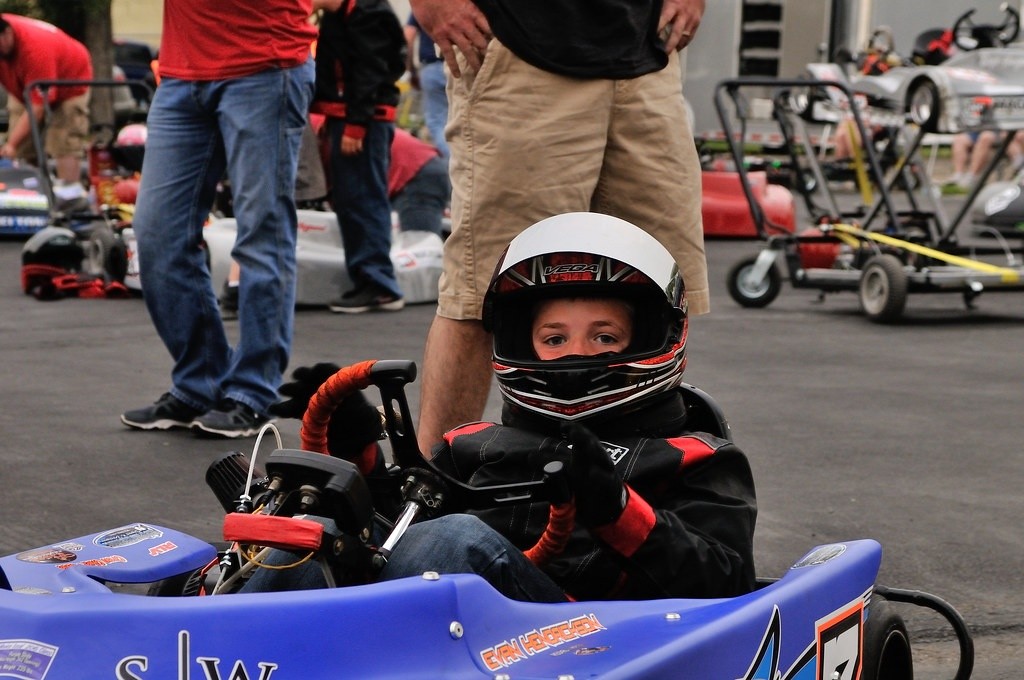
left=268, top=361, right=383, bottom=454
left=545, top=430, right=630, bottom=532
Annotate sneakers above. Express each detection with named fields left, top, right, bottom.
left=330, top=284, right=404, bottom=313
left=189, top=397, right=276, bottom=437
left=121, top=391, right=206, bottom=429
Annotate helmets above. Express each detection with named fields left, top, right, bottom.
left=482, top=211, right=688, bottom=430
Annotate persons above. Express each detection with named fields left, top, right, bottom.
left=835, top=47, right=1024, bottom=191
left=410, top=0, right=704, bottom=462
left=308, top=111, right=453, bottom=241
left=404, top=12, right=450, bottom=171
left=120, top=0, right=343, bottom=438
left=0, top=14, right=93, bottom=184
left=307, top=0, right=404, bottom=313
left=269, top=212, right=757, bottom=604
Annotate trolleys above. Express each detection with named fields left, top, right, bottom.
left=713, top=71, right=1024, bottom=327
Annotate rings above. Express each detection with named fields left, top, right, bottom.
left=682, top=31, right=690, bottom=36
